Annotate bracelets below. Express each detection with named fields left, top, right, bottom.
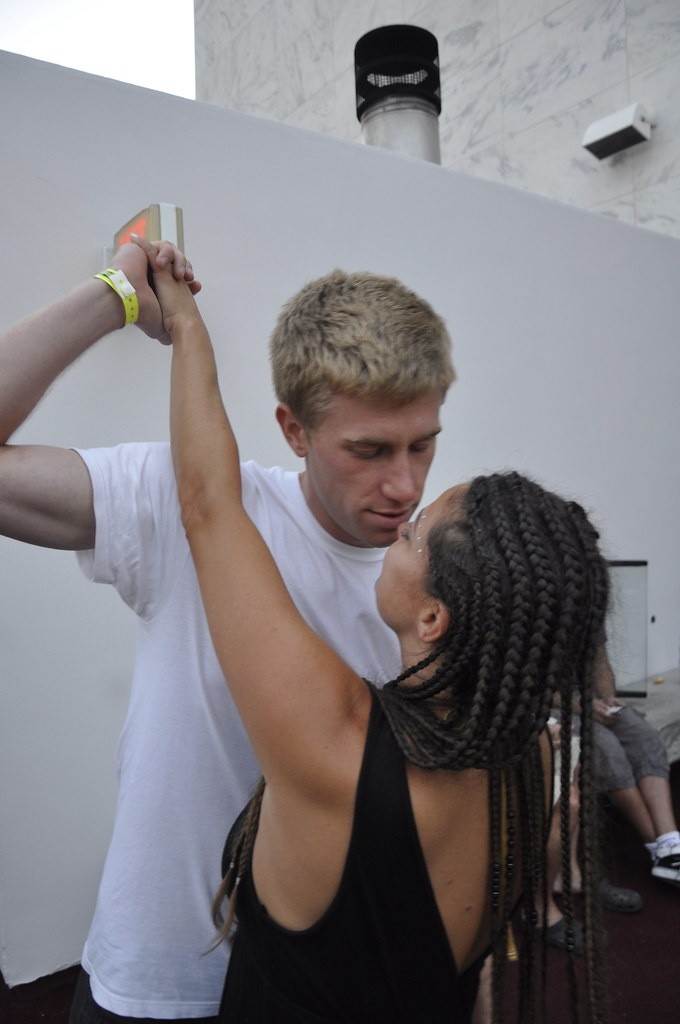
left=93, top=270, right=139, bottom=330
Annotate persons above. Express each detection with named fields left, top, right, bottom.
left=128, top=234, right=680, bottom=1024
left=0, top=242, right=456, bottom=1024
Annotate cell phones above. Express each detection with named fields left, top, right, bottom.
left=608, top=705, right=625, bottom=715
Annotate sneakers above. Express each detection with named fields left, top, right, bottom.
left=651, top=845, right=680, bottom=881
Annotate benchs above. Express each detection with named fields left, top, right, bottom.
left=619, top=666, right=679, bottom=763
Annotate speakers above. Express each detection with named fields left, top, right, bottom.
left=582, top=101, right=652, bottom=161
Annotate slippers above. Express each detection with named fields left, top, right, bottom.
left=574, top=878, right=645, bottom=913
left=538, top=916, right=585, bottom=956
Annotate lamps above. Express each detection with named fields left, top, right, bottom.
left=581, top=102, right=657, bottom=161
